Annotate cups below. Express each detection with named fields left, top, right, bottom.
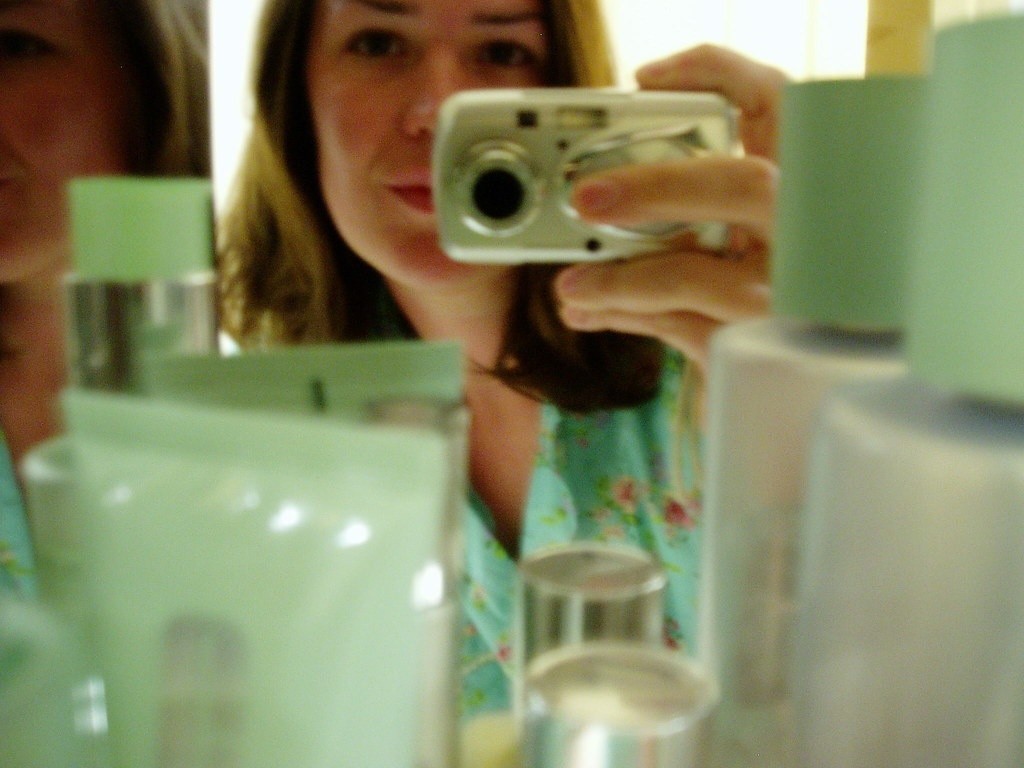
left=520, top=643, right=718, bottom=768
left=514, top=548, right=665, bottom=747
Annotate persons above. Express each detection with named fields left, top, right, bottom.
left=0, top=0, right=210, bottom=599
left=222, top=0, right=798, bottom=725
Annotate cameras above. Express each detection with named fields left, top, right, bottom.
left=427, top=85, right=745, bottom=265
